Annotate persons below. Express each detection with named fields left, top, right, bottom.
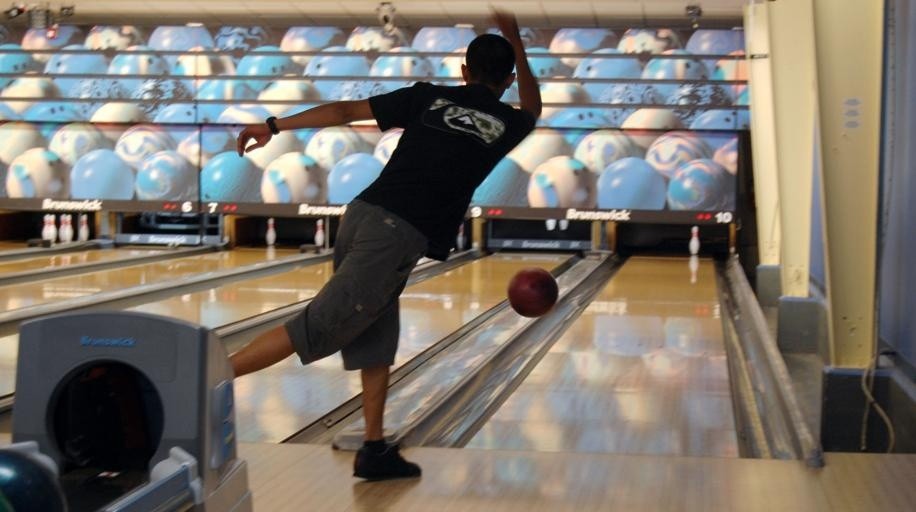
left=209, top=4, right=542, bottom=479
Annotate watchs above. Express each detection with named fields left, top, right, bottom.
left=265, top=115, right=279, bottom=134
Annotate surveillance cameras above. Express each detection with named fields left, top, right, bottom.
left=378, top=5, right=394, bottom=25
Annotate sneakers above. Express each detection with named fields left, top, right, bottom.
left=355, top=440, right=421, bottom=480
